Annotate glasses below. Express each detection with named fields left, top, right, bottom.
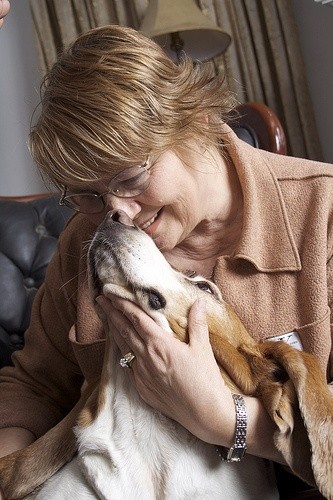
left=58, top=153, right=155, bottom=217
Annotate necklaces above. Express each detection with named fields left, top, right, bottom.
left=184, top=269, right=198, bottom=277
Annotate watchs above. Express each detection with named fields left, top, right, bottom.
left=215, top=394, right=248, bottom=463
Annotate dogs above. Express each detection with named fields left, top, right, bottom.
left=0, top=209, right=333, bottom=500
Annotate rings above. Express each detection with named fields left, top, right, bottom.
left=119, top=353, right=136, bottom=368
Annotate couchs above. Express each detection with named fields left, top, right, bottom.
left=0, top=103, right=288, bottom=367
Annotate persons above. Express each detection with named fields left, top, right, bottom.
left=0, top=25, right=333, bottom=500
left=0, top=0, right=11, bottom=27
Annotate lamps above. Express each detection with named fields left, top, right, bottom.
left=137, top=0, right=233, bottom=66
